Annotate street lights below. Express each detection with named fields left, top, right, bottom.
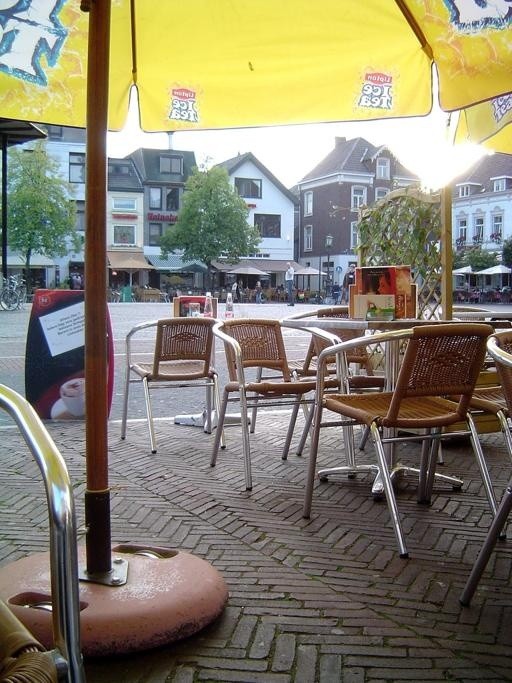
left=325, top=233, right=334, bottom=296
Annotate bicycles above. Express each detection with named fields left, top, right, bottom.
left=0, top=274, right=27, bottom=311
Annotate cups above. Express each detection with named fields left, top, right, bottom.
left=61, top=381, right=93, bottom=417
left=188, top=303, right=200, bottom=320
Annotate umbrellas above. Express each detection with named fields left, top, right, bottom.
left=0, top=0, right=511, bottom=563
left=107, top=256, right=329, bottom=290
left=424, top=262, right=512, bottom=289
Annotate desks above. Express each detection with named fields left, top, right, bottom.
left=173, top=315, right=251, bottom=434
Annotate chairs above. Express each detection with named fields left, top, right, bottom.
left=302, top=321, right=507, bottom=559
left=460, top=327, right=512, bottom=605
left=280, top=306, right=510, bottom=501
left=210, top=317, right=358, bottom=490
left=122, top=318, right=226, bottom=453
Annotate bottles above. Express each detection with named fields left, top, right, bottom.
left=204, top=292, right=213, bottom=318
left=225, top=293, right=234, bottom=321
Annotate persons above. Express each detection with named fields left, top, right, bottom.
left=229, top=279, right=284, bottom=303
left=67, top=270, right=84, bottom=290
left=319, top=262, right=357, bottom=305
left=284, top=261, right=295, bottom=306
left=453, top=279, right=511, bottom=305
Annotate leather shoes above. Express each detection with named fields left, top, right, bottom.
left=288, top=303, right=294, bottom=306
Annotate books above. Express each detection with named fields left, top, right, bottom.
left=345, top=262, right=418, bottom=320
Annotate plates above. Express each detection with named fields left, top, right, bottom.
left=51, top=400, right=89, bottom=420
